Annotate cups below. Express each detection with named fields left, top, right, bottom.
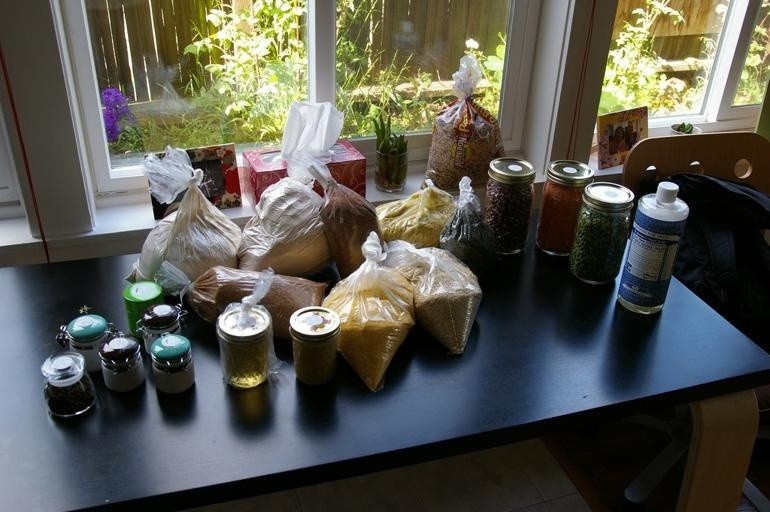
left=375, top=148, right=407, bottom=193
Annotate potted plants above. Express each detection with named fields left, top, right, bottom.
left=369, top=112, right=410, bottom=193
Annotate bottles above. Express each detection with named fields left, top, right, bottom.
left=616, top=180, right=691, bottom=315
left=41, top=280, right=195, bottom=419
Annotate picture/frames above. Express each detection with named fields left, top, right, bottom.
left=145, top=142, right=243, bottom=221
left=596, top=105, right=649, bottom=170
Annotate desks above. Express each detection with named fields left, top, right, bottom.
left=0, top=199, right=769, bottom=512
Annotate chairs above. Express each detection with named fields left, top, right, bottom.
left=623, top=132, right=770, bottom=512
